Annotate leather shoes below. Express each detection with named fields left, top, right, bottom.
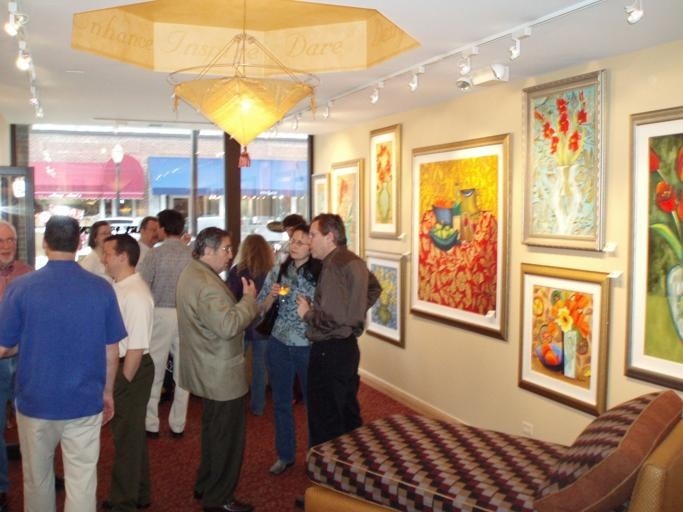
left=100, top=500, right=150, bottom=510
left=203, top=499, right=253, bottom=512
left=295, top=494, right=304, bottom=509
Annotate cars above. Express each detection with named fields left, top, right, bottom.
left=82, top=214, right=289, bottom=250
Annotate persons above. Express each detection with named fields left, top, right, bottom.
left=177, top=227, right=262, bottom=511
left=0, top=215, right=128, bottom=511
left=79, top=221, right=111, bottom=273
left=1, top=221, right=64, bottom=512
left=225, top=234, right=277, bottom=417
left=296, top=213, right=383, bottom=511
left=257, top=225, right=318, bottom=474
left=137, top=210, right=304, bottom=437
left=102, top=233, right=155, bottom=509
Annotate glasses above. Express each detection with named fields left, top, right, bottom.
left=288, top=237, right=309, bottom=246
left=219, top=246, right=233, bottom=254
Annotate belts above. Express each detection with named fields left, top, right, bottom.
left=118, top=353, right=150, bottom=363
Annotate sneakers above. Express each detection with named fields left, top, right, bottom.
left=269, top=460, right=294, bottom=475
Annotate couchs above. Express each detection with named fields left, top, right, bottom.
left=296, top=388, right=682, bottom=512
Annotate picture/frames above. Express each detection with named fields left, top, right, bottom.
left=366, top=121, right=402, bottom=243
left=515, top=259, right=611, bottom=418
left=408, top=131, right=513, bottom=343
left=622, top=105, right=682, bottom=387
left=306, top=173, right=329, bottom=221
left=360, top=247, right=408, bottom=352
left=328, top=157, right=366, bottom=264
left=519, top=67, right=608, bottom=257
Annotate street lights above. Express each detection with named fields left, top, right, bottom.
left=110, top=141, right=125, bottom=216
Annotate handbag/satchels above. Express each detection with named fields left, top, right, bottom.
left=255, top=264, right=282, bottom=336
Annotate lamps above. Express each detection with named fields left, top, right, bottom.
left=0, top=0, right=55, bottom=126
left=165, top=0, right=317, bottom=170
left=255, top=0, right=648, bottom=140
